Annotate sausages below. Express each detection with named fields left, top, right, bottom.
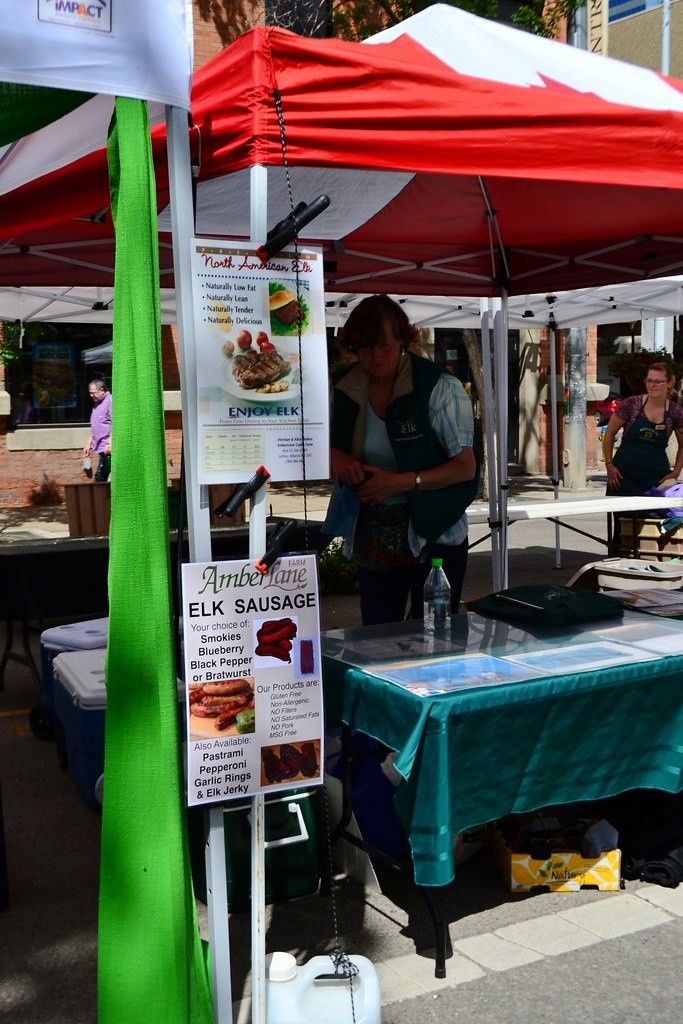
left=189, top=679, right=255, bottom=730
left=255, top=618, right=297, bottom=663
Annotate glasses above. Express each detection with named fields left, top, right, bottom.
left=644, top=377, right=669, bottom=385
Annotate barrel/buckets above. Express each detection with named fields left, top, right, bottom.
left=193, top=787, right=321, bottom=916
left=265, top=952, right=382, bottom=1023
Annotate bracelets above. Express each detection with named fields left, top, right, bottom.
left=605, top=462, right=613, bottom=465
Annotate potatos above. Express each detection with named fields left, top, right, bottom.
left=222, top=329, right=275, bottom=356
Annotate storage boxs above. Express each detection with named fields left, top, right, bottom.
left=564, top=557, right=683, bottom=590
left=170, top=473, right=249, bottom=530
left=506, top=814, right=626, bottom=898
left=615, top=516, right=683, bottom=563
left=57, top=480, right=113, bottom=536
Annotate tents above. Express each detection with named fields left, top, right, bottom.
left=0, top=0, right=683, bottom=1024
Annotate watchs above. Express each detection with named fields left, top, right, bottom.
left=413, top=471, right=421, bottom=490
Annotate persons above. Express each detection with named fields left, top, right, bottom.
left=603, top=364, right=683, bottom=559
left=329, top=298, right=483, bottom=626
left=79, top=379, right=113, bottom=483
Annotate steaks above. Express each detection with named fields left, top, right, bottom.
left=233, top=349, right=288, bottom=388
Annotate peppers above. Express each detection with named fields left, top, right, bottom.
left=235, top=708, right=254, bottom=734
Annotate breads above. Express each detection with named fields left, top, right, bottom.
left=268, top=289, right=295, bottom=310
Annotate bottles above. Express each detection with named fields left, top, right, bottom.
left=423, top=557, right=452, bottom=633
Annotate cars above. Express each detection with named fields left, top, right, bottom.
left=594, top=392, right=624, bottom=426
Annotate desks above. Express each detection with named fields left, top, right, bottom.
left=0, top=519, right=327, bottom=692
left=319, top=603, right=683, bottom=983
left=464, top=495, right=683, bottom=592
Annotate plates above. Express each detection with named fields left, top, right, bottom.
left=212, top=345, right=312, bottom=402
left=269, top=280, right=312, bottom=336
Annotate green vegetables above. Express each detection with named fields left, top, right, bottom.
left=268, top=281, right=309, bottom=335
